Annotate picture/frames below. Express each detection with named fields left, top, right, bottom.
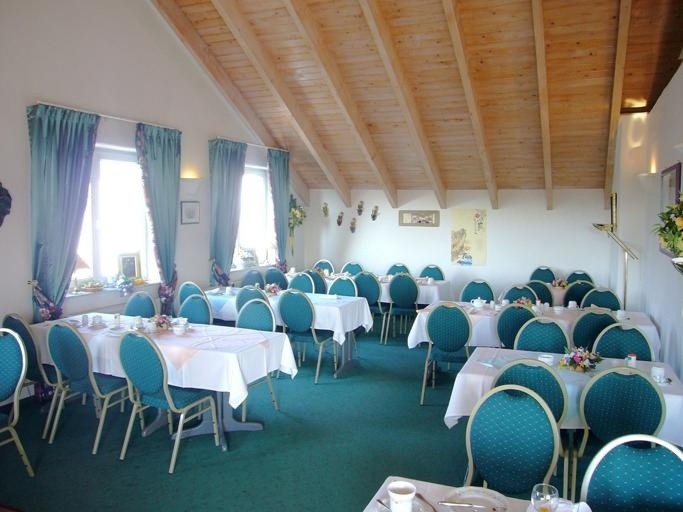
left=658, top=162, right=681, bottom=259
left=399, top=210, right=440, bottom=227
left=180, top=200, right=201, bottom=224
left=118, top=253, right=141, bottom=279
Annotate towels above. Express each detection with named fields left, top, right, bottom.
left=527, top=498, right=591, bottom=512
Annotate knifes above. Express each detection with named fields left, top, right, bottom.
left=438, top=501, right=487, bottom=508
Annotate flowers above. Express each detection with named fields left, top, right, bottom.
left=148, top=312, right=174, bottom=332
left=648, top=188, right=683, bottom=258
left=288, top=195, right=306, bottom=255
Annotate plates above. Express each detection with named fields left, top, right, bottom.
left=527, top=497, right=572, bottom=512
left=375, top=495, right=435, bottom=512
left=443, top=485, right=508, bottom=512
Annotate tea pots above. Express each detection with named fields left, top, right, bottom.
left=471, top=297, right=487, bottom=308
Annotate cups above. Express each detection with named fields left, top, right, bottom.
left=530, top=483, right=559, bottom=512
left=82, top=315, right=87, bottom=327
left=428, top=278, right=434, bottom=285
left=173, top=325, right=184, bottom=336
left=290, top=267, right=295, bottom=274
left=225, top=287, right=231, bottom=295
left=553, top=306, right=564, bottom=316
left=490, top=301, right=495, bottom=309
left=617, top=310, right=625, bottom=320
left=379, top=276, right=383, bottom=283
left=651, top=366, right=666, bottom=383
left=114, top=313, right=120, bottom=327
left=93, top=316, right=103, bottom=326
left=501, top=299, right=509, bottom=307
left=135, top=316, right=142, bottom=327
left=388, top=481, right=417, bottom=512
left=627, top=355, right=637, bottom=367
left=569, top=300, right=577, bottom=310
left=536, top=301, right=541, bottom=308
left=538, top=355, right=553, bottom=367
left=324, top=269, right=329, bottom=277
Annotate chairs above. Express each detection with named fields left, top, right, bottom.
left=579, top=432, right=683, bottom=511
left=119, top=330, right=221, bottom=474
left=483, top=359, right=569, bottom=500
left=461, top=385, right=562, bottom=502
left=0, top=312, right=87, bottom=441
left=235, top=299, right=279, bottom=422
left=0, top=328, right=36, bottom=478
left=570, top=366, right=667, bottom=504
left=123, top=292, right=156, bottom=318
left=46, top=321, right=146, bottom=455
